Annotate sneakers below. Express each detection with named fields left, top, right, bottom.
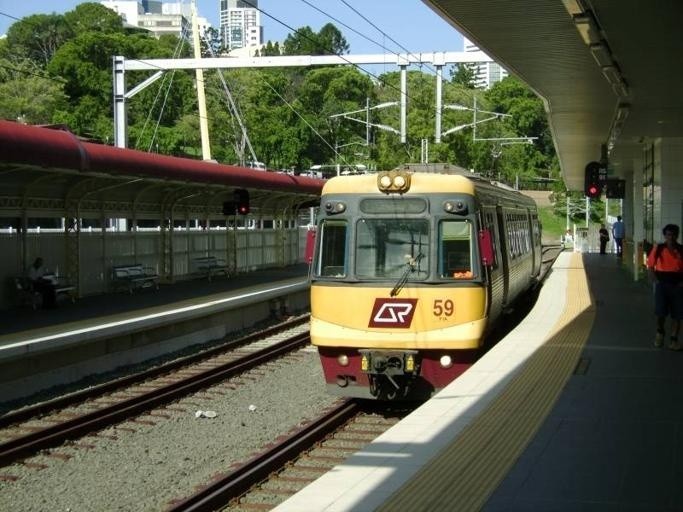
left=655, top=333, right=663, bottom=347
left=669, top=340, right=681, bottom=350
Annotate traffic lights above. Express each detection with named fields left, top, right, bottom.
left=236, top=189, right=250, bottom=215
left=585, top=161, right=604, bottom=198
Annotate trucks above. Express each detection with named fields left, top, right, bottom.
left=300, top=165, right=366, bottom=179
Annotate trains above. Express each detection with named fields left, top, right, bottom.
left=303, top=163, right=542, bottom=400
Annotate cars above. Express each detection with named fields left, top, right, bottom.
left=274, top=169, right=291, bottom=174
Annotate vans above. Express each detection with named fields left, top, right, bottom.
left=250, top=162, right=266, bottom=172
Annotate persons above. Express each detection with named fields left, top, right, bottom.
left=22, top=257, right=58, bottom=310
left=645, top=224, right=683, bottom=351
left=599, top=224, right=609, bottom=254
left=612, top=216, right=625, bottom=256
